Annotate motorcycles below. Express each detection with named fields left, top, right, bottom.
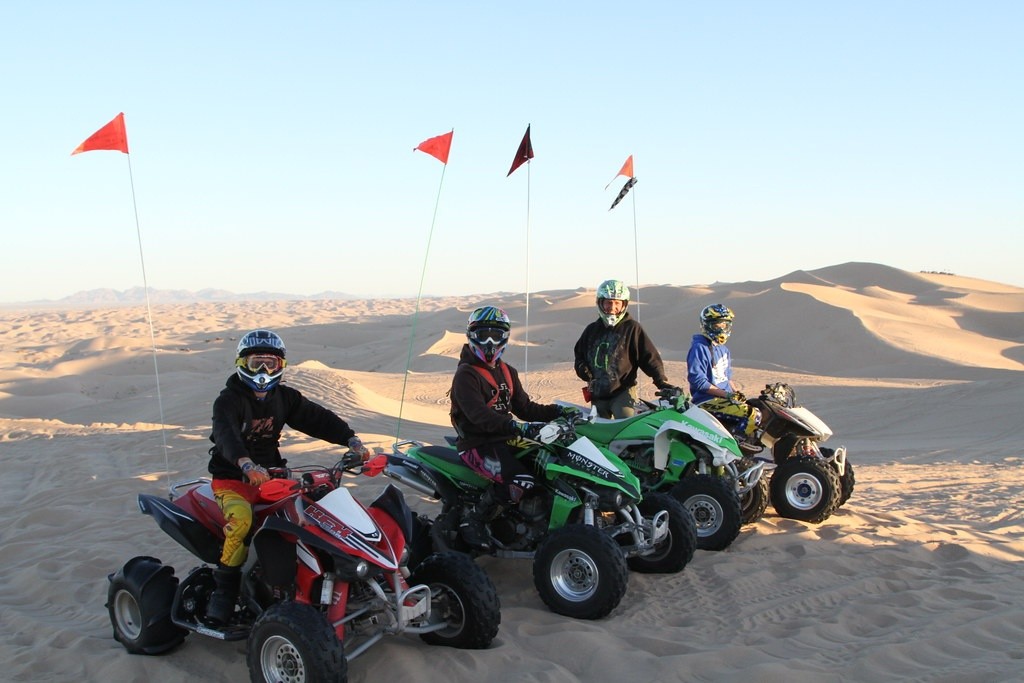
left=635, top=379, right=857, bottom=524
left=378, top=412, right=698, bottom=620
left=106, top=448, right=501, bottom=683
left=533, top=384, right=775, bottom=551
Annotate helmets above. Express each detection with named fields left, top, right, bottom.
left=596, top=280, right=631, bottom=327
left=235, top=330, right=285, bottom=392
left=700, top=303, right=735, bottom=345
left=467, top=307, right=510, bottom=364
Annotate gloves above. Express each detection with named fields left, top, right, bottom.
left=242, top=462, right=271, bottom=487
left=557, top=406, right=581, bottom=419
left=511, top=419, right=548, bottom=447
left=346, top=436, right=371, bottom=463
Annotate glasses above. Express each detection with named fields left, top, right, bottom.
left=246, top=354, right=280, bottom=374
left=474, top=329, right=506, bottom=346
left=708, top=321, right=732, bottom=332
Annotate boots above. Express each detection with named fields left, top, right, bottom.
left=459, top=485, right=506, bottom=549
left=205, top=571, right=244, bottom=625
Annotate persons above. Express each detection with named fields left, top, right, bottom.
left=207, top=330, right=371, bottom=622
left=449, top=307, right=582, bottom=549
left=686, top=303, right=762, bottom=442
left=574, top=280, right=675, bottom=419
